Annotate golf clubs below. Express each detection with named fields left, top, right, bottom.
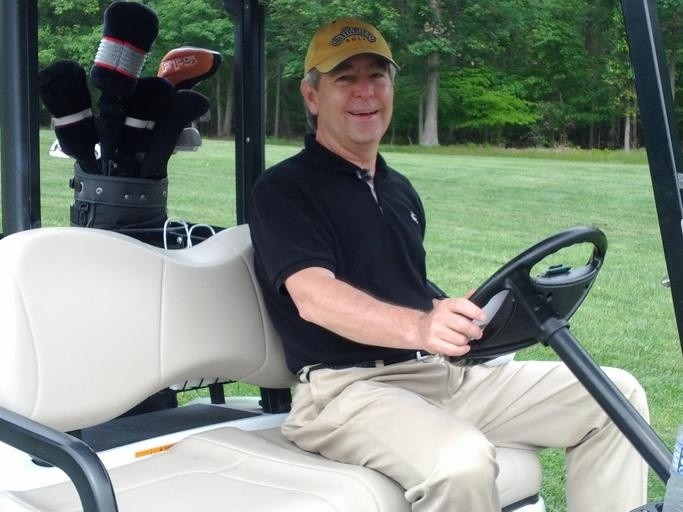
left=40, top=1, right=222, bottom=180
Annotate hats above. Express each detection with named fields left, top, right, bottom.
left=303, top=19, right=402, bottom=74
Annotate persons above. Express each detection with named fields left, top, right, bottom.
left=245, top=16, right=651, bottom=512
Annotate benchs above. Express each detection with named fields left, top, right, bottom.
left=0, top=223, right=540, bottom=510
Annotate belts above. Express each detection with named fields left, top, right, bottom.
left=298, top=348, right=431, bottom=385
